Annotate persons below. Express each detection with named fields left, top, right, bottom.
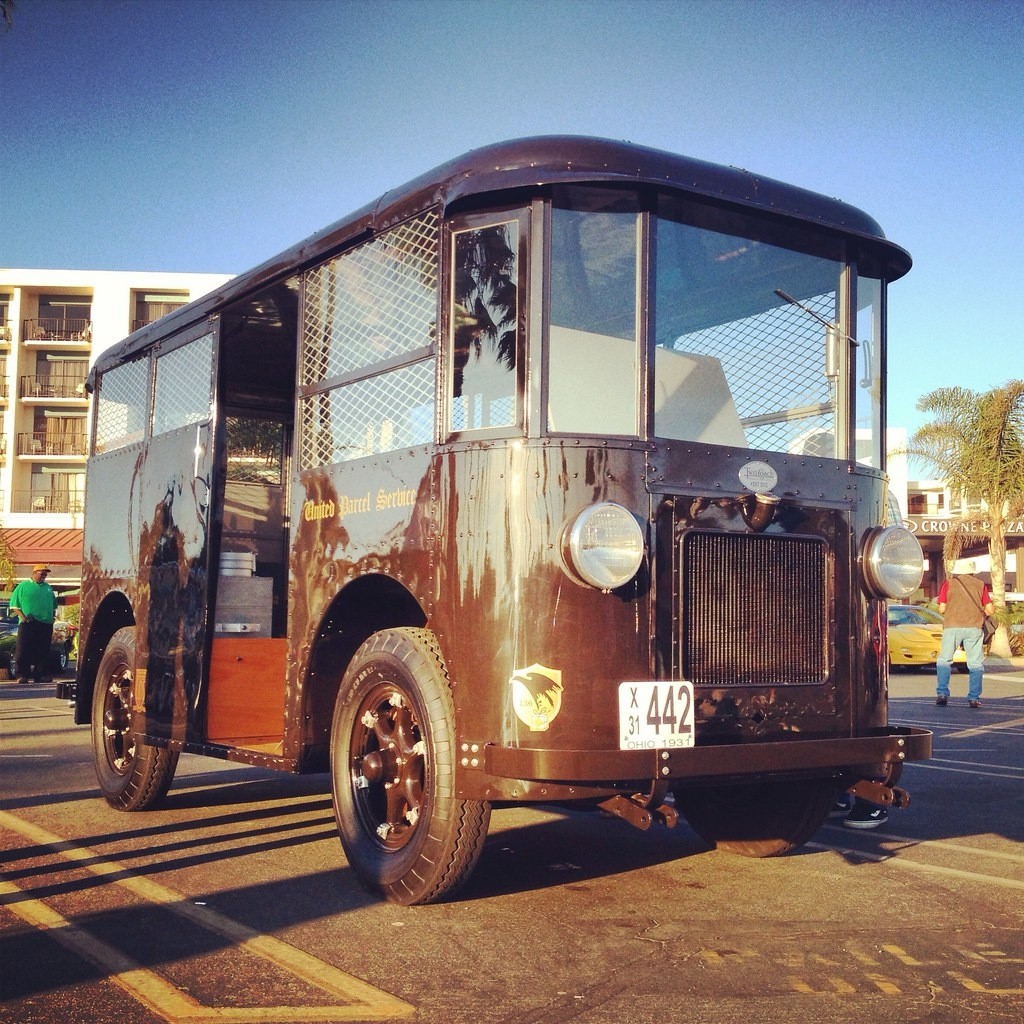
left=9, top=564, right=58, bottom=683
left=936, top=558, right=995, bottom=708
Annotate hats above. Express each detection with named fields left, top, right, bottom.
left=33, top=565, right=52, bottom=573
left=949, top=559, right=981, bottom=576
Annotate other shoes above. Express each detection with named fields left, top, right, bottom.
left=34, top=675, right=52, bottom=682
left=936, top=695, right=948, bottom=705
left=969, top=698, right=981, bottom=708
left=17, top=676, right=28, bottom=684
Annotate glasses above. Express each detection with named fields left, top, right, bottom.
left=37, top=572, right=47, bottom=577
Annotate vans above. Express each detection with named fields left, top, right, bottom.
left=54, top=136, right=933, bottom=907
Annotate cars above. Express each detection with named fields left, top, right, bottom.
left=988, top=592, right=1024, bottom=607
left=887, top=605, right=988, bottom=674
left=0, top=612, right=79, bottom=679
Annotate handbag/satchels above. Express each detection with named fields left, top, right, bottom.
left=983, top=615, right=999, bottom=643
